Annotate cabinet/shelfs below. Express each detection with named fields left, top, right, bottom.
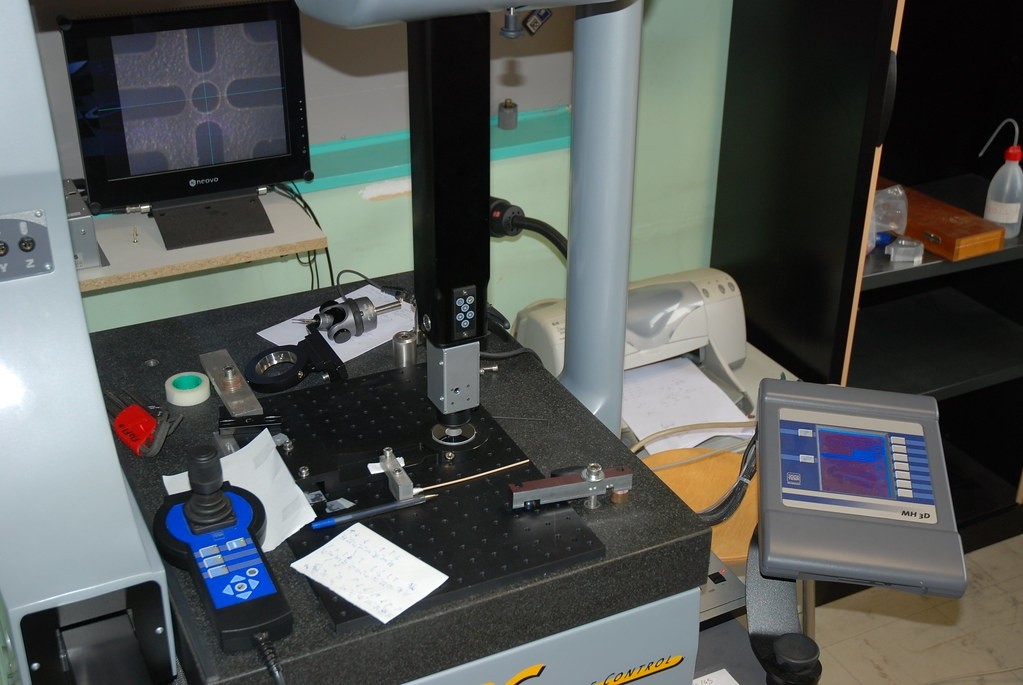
left=708, top=1, right=1023, bottom=610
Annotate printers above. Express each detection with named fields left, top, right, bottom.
left=515, top=268, right=798, bottom=452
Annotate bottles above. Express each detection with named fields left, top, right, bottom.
left=984, top=147, right=1023, bottom=239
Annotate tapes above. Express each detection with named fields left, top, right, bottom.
left=165, top=371, right=211, bottom=407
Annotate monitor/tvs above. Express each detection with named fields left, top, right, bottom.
left=58, top=0, right=313, bottom=210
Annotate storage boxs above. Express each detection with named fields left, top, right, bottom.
left=877, top=176, right=1006, bottom=262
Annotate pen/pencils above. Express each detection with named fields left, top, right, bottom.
left=311, top=492, right=440, bottom=531
